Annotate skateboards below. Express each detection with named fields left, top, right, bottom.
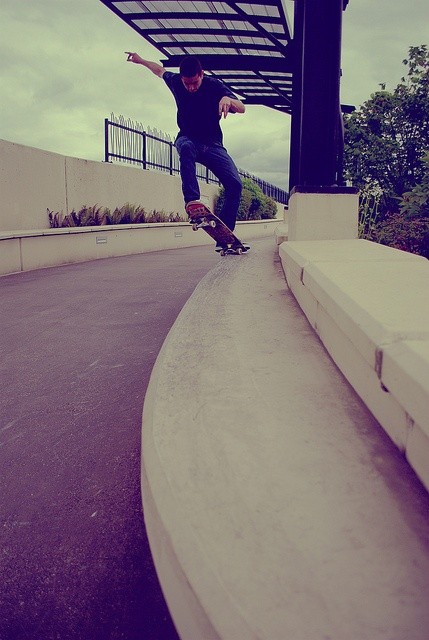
left=185, top=200, right=247, bottom=256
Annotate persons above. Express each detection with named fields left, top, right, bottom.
left=124, top=48, right=252, bottom=256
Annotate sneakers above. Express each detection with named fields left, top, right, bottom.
left=214, top=241, right=251, bottom=253
